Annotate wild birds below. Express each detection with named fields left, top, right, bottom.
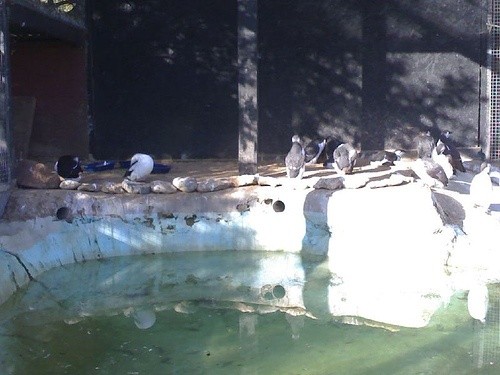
left=369, top=148, right=406, bottom=164
left=54, top=155, right=83, bottom=181
left=417, top=129, right=466, bottom=188
left=468, top=284, right=489, bottom=323
left=304, top=136, right=329, bottom=164
left=332, top=143, right=356, bottom=174
left=122, top=152, right=154, bottom=181
left=470, top=162, right=493, bottom=213
left=285, top=135, right=306, bottom=179
left=420, top=181, right=467, bottom=275
